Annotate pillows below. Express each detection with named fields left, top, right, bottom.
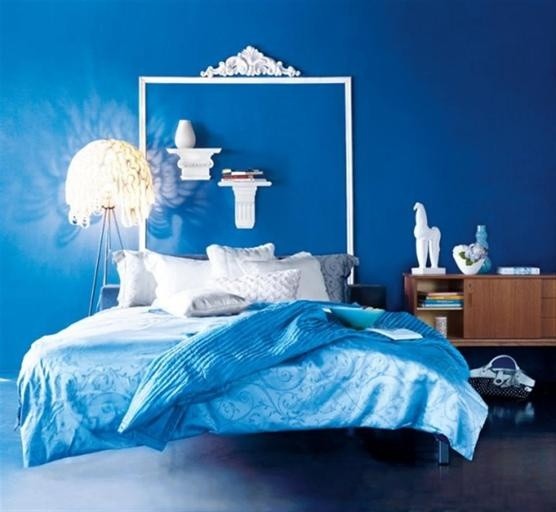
left=113, top=243, right=360, bottom=319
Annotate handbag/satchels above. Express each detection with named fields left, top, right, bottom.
left=468, top=354, right=536, bottom=400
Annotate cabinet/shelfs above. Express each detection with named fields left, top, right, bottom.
left=404, top=273, right=556, bottom=346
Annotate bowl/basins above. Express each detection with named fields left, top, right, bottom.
left=327, top=307, right=385, bottom=330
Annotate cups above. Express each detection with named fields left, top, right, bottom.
left=434, top=316, right=448, bottom=339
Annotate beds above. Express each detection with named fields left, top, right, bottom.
left=14, top=244, right=490, bottom=468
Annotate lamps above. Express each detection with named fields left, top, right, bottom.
left=65, top=139, right=153, bottom=317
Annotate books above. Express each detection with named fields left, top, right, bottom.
left=417, top=292, right=464, bottom=308
left=496, top=266, right=541, bottom=275
left=220, top=167, right=268, bottom=183
